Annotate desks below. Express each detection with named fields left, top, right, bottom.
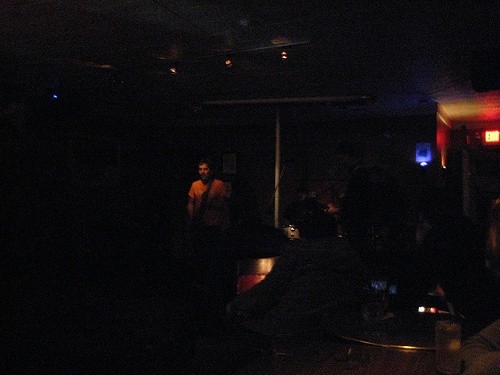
left=227, top=339, right=444, bottom=375
left=325, top=308, right=464, bottom=350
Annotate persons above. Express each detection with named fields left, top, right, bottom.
left=186, top=155, right=227, bottom=287
left=282, top=184, right=328, bottom=241
left=323, top=139, right=389, bottom=261
left=414, top=144, right=500, bottom=375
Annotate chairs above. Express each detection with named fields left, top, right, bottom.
left=184, top=255, right=283, bottom=364
